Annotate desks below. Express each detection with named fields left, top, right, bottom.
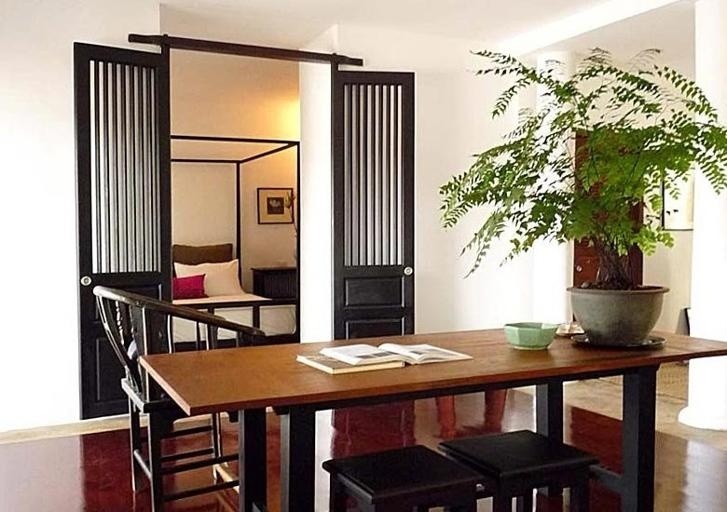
left=137, top=320, right=727, bottom=511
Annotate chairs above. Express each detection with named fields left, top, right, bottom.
left=91, top=284, right=268, bottom=512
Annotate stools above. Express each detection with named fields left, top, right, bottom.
left=316, top=442, right=481, bottom=511
left=436, top=428, right=602, bottom=510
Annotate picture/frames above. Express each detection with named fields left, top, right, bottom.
left=255, top=186, right=294, bottom=225
left=660, top=161, right=692, bottom=233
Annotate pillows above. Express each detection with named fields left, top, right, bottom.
left=171, top=272, right=209, bottom=299
left=174, top=259, right=249, bottom=299
left=171, top=242, right=233, bottom=277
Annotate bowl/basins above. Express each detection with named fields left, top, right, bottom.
left=504, top=321, right=559, bottom=350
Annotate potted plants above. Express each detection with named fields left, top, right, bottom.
left=433, top=43, right=727, bottom=353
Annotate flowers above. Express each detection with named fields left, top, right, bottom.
left=282, top=190, right=298, bottom=238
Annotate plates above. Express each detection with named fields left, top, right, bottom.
left=556, top=321, right=585, bottom=336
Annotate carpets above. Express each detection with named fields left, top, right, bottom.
left=601, top=360, right=691, bottom=403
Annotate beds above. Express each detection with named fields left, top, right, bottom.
left=169, top=133, right=300, bottom=351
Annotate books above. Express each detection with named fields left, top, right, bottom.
left=295, top=342, right=474, bottom=374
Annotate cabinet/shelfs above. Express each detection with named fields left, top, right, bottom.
left=249, top=265, right=298, bottom=302
